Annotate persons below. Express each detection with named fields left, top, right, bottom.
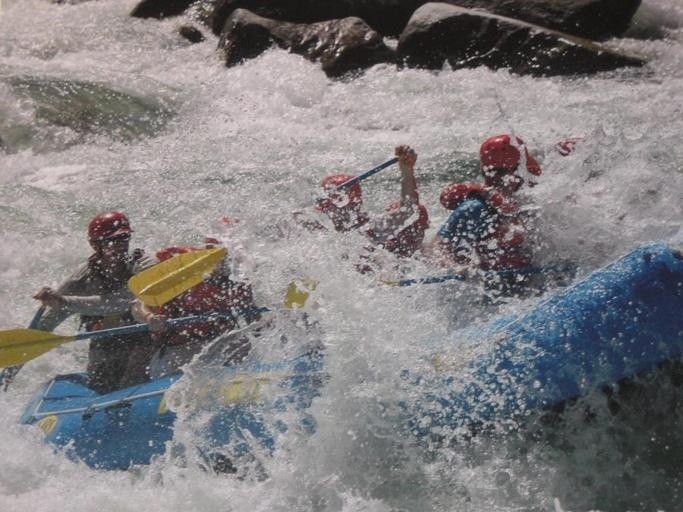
left=24, top=210, right=158, bottom=399
left=430, top=131, right=570, bottom=294
left=121, top=235, right=248, bottom=390
left=290, top=146, right=425, bottom=272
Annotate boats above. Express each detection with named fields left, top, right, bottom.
left=16, top=242, right=683, bottom=485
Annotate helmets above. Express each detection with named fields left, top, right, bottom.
left=481, top=136, right=542, bottom=177
left=318, top=175, right=362, bottom=210
left=386, top=202, right=428, bottom=229
left=88, top=212, right=132, bottom=242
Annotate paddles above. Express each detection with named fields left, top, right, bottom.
left=285, top=261, right=582, bottom=312
left=0, top=302, right=283, bottom=369
left=128, top=151, right=407, bottom=308
